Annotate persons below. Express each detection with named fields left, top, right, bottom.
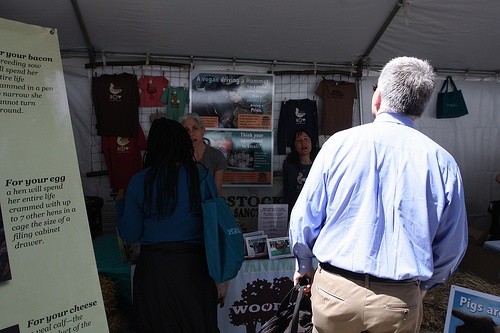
left=283, top=129, right=320, bottom=214
left=115, top=117, right=227, bottom=333
left=289, top=56, right=469, bottom=333
left=227, top=89, right=252, bottom=119
left=178, top=113, right=227, bottom=200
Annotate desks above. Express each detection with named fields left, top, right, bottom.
left=217, top=258, right=318, bottom=333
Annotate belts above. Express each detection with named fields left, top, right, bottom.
left=319, top=262, right=416, bottom=284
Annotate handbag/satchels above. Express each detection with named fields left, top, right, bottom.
left=257, top=279, right=313, bottom=333
left=436, top=75, right=469, bottom=119
left=200, top=166, right=245, bottom=283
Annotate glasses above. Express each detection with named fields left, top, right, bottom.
left=373, top=85, right=378, bottom=92
